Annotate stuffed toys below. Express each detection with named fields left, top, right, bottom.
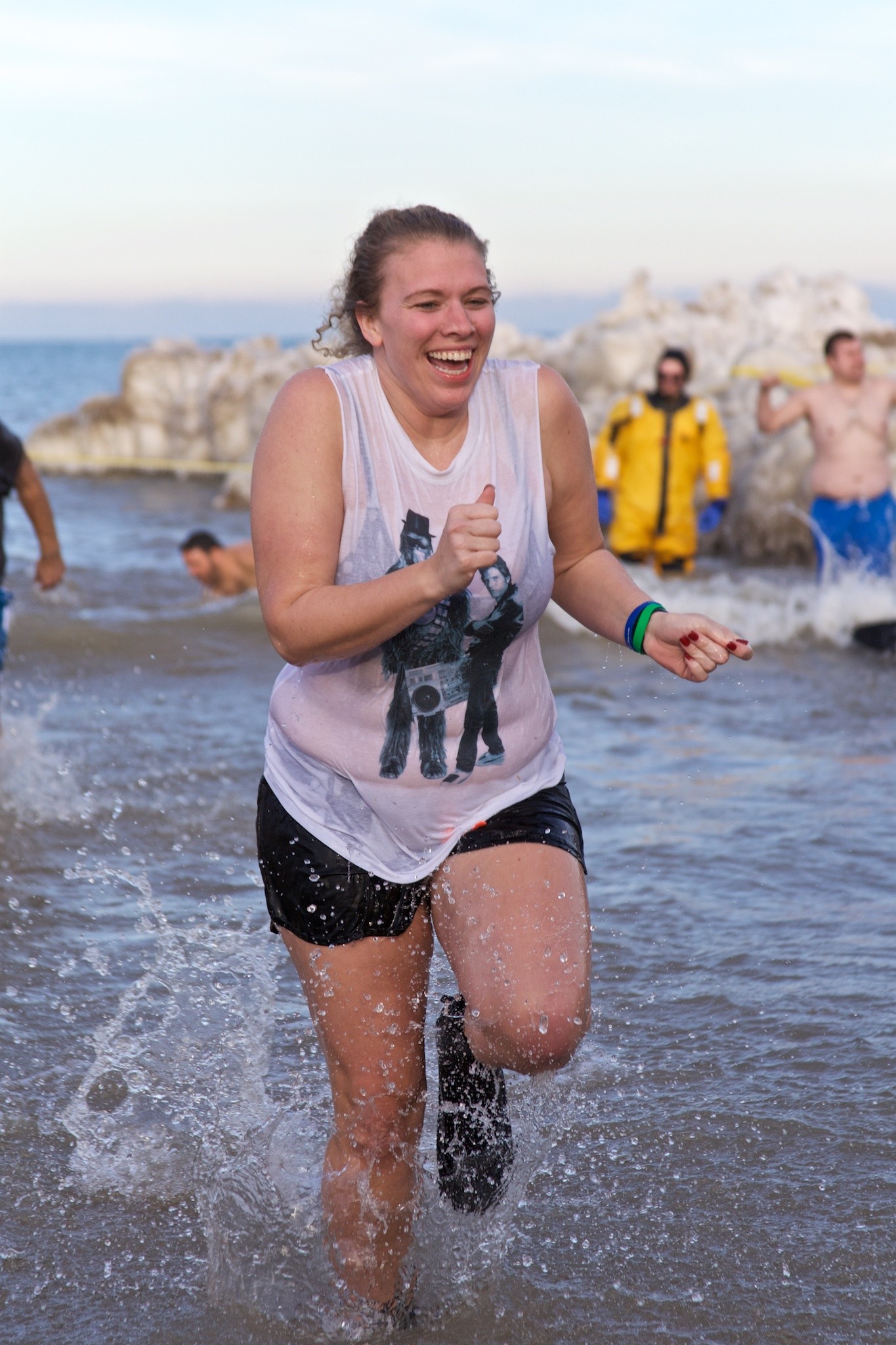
left=380, top=510, right=470, bottom=780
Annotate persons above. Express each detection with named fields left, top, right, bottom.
left=249, top=207, right=754, bottom=1330
left=756, top=331, right=896, bottom=582
left=594, top=348, right=730, bottom=577
left=0, top=422, right=64, bottom=665
left=440, top=555, right=524, bottom=786
left=182, top=531, right=257, bottom=596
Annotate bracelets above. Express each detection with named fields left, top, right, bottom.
left=624, top=601, right=667, bottom=655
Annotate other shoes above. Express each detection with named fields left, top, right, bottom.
left=436, top=992, right=515, bottom=1211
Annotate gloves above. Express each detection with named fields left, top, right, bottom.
left=598, top=492, right=612, bottom=527
left=698, top=503, right=724, bottom=530
